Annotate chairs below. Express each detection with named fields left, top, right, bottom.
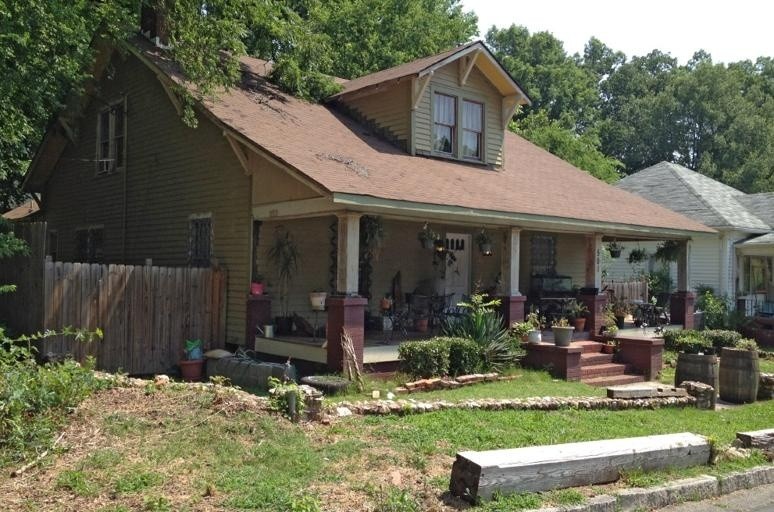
left=391, top=273, right=454, bottom=336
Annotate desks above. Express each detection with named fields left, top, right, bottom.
left=309, top=309, right=326, bottom=341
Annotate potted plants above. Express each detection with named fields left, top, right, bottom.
left=307, top=286, right=328, bottom=312
left=475, top=231, right=495, bottom=255
left=176, top=337, right=205, bottom=380
left=605, top=242, right=624, bottom=259
left=263, top=231, right=304, bottom=335
left=379, top=311, right=395, bottom=333
left=524, top=305, right=544, bottom=342
left=417, top=222, right=440, bottom=249
left=570, top=299, right=589, bottom=332
left=551, top=317, right=576, bottom=345
left=602, top=324, right=617, bottom=354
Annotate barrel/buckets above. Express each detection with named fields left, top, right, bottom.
left=718, top=346, right=760, bottom=404
left=380, top=315, right=394, bottom=332
left=614, top=315, right=624, bottom=330
left=673, top=350, right=718, bottom=411
left=307, top=291, right=327, bottom=311
left=415, top=318, right=429, bottom=332
left=251, top=279, right=266, bottom=296
